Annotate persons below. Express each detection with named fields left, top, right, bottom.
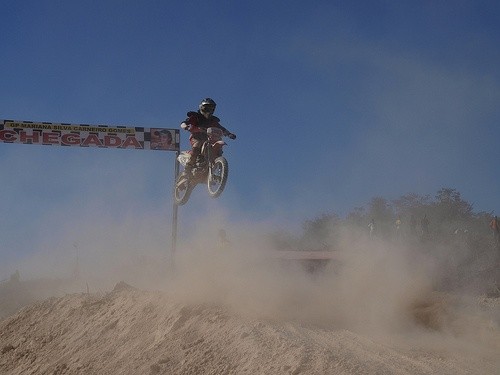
left=488, top=216, right=500, bottom=241
left=180, top=98, right=237, bottom=178
left=367, top=214, right=429, bottom=242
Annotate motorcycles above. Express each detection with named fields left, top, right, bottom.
left=173, top=120, right=228, bottom=204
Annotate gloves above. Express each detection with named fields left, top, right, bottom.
left=226, top=132, right=236, bottom=139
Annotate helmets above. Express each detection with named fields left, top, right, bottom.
left=198, top=98, right=218, bottom=117
